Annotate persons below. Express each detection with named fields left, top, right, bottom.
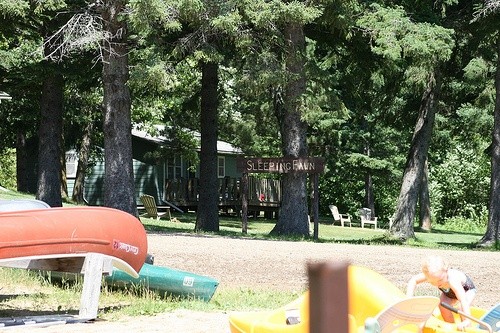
left=260, top=191, right=265, bottom=201
left=406, top=254, right=477, bottom=331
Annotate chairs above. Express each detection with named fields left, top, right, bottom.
left=478, top=303, right=500, bottom=333
left=355, top=208, right=378, bottom=229
left=139, top=195, right=171, bottom=222
left=308, top=215, right=321, bottom=235
left=329, top=205, right=353, bottom=227
left=376, top=296, right=440, bottom=333
